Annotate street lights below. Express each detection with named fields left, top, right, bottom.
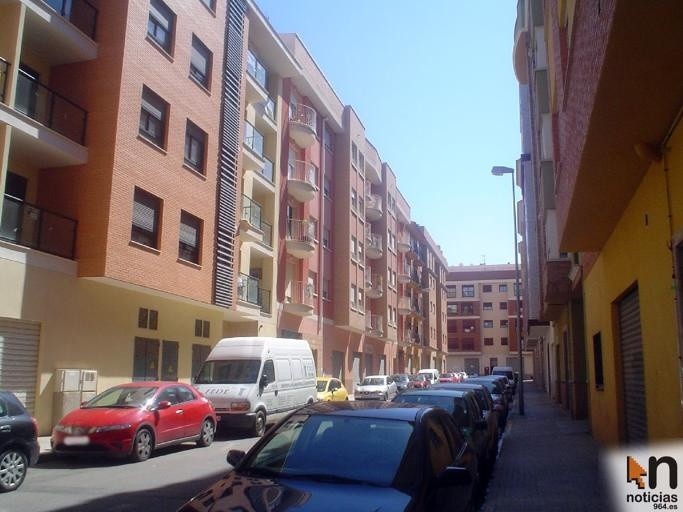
left=491, top=165, right=525, bottom=417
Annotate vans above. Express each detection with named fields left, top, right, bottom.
left=191, top=337, right=320, bottom=438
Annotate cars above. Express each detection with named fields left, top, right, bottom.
left=48, top=380, right=219, bottom=462
left=179, top=366, right=518, bottom=511
left=0, top=389, right=42, bottom=494
